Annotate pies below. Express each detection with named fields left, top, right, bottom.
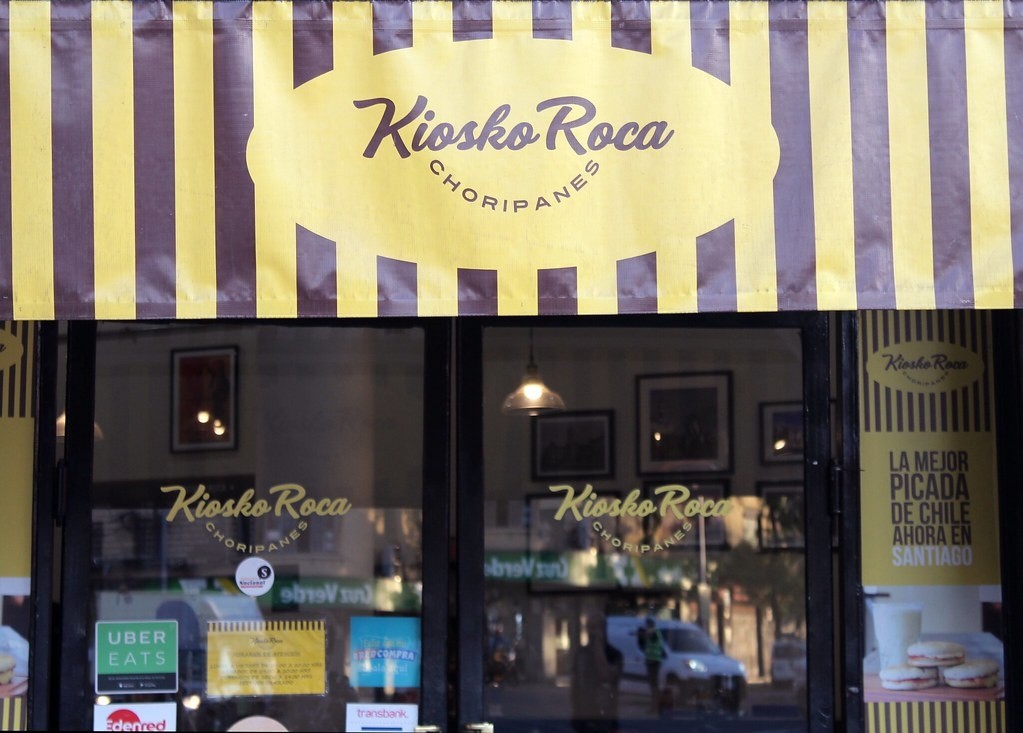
left=878, top=640, right=1001, bottom=691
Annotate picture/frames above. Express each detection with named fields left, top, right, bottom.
left=166, top=347, right=244, bottom=454
left=643, top=478, right=733, bottom=552
left=757, top=395, right=805, bottom=467
left=633, top=368, right=737, bottom=480
left=521, top=487, right=623, bottom=599
left=528, top=409, right=620, bottom=485
left=751, top=477, right=810, bottom=556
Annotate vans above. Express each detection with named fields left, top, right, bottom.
left=605, top=613, right=748, bottom=719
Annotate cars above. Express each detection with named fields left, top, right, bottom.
left=769, top=636, right=807, bottom=690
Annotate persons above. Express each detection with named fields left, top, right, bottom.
left=568, top=612, right=625, bottom=728
left=637, top=620, right=668, bottom=712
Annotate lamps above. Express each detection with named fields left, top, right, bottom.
left=501, top=326, right=567, bottom=419
